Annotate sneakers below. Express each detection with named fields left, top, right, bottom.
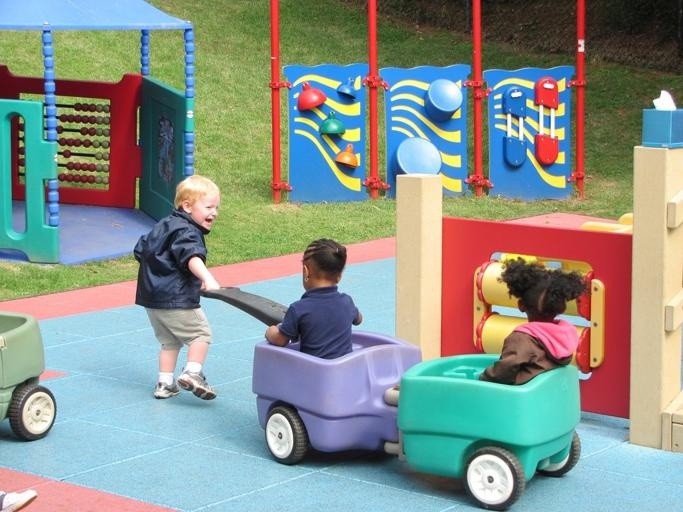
left=177, top=368, right=217, bottom=400
left=0, top=489, right=37, bottom=512
left=154, top=377, right=180, bottom=398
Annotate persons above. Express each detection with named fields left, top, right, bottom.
left=478, top=257, right=591, bottom=385
left=264, top=237, right=363, bottom=359
left=131, top=174, right=222, bottom=401
